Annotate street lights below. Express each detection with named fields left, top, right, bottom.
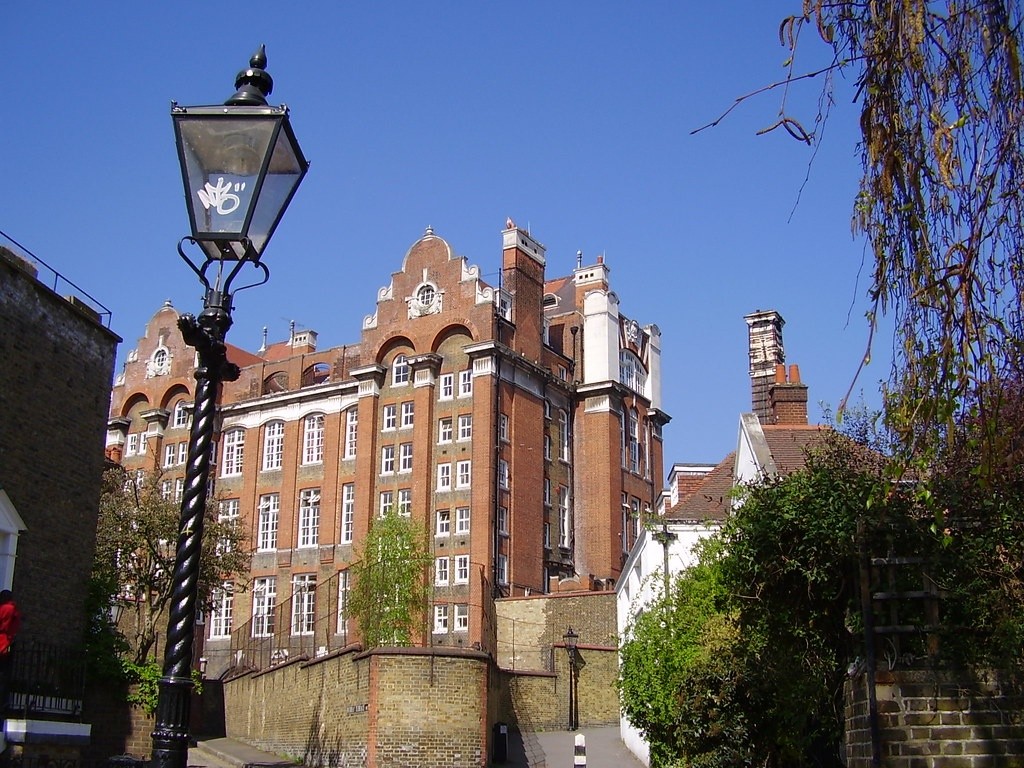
left=561, top=624, right=579, bottom=731
left=144, top=44, right=315, bottom=768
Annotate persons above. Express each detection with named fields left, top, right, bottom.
left=0, top=589, right=21, bottom=731
